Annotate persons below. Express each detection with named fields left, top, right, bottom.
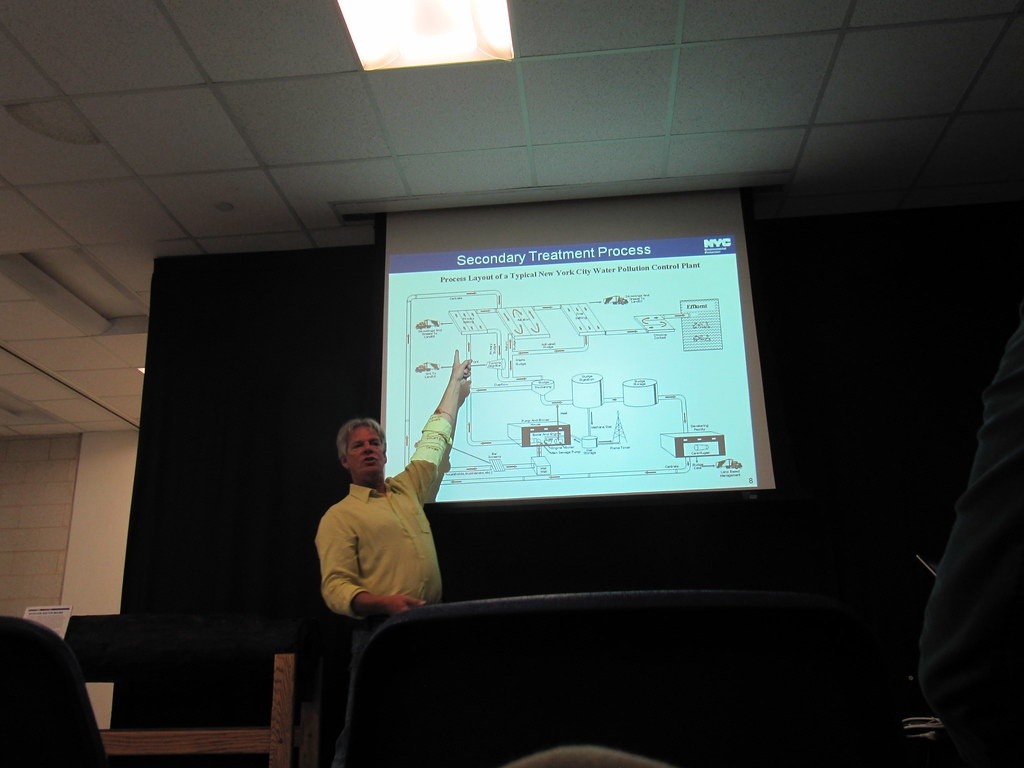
left=914, top=317, right=1024, bottom=768
left=313, top=349, right=474, bottom=767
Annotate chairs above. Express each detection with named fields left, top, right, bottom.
left=323, top=587, right=905, bottom=768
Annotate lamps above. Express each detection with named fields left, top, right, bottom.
left=336, top=0, right=516, bottom=73
